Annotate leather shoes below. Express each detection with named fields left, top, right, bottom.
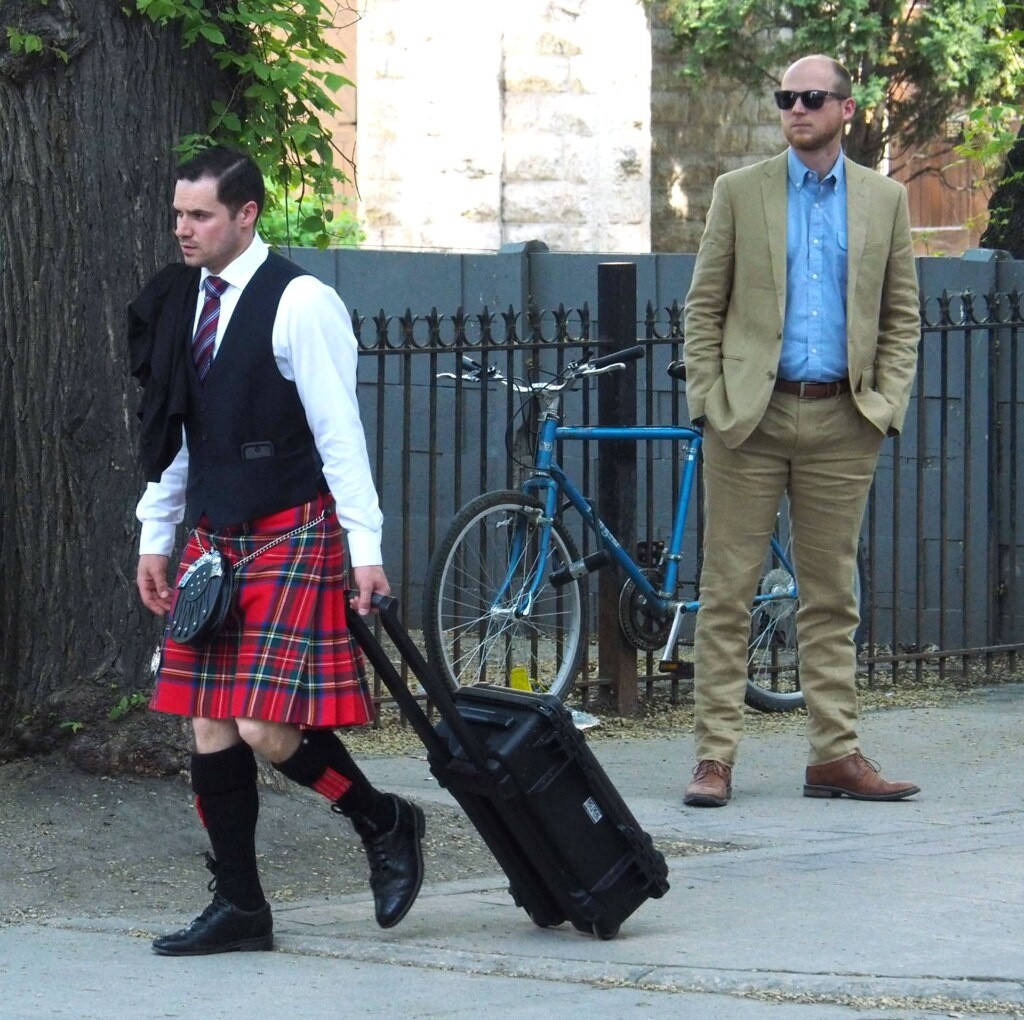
left=803, top=747, right=921, bottom=801
left=682, top=760, right=732, bottom=805
left=151, top=892, right=274, bottom=955
left=360, top=793, right=426, bottom=929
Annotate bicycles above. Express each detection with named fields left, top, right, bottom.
left=419, top=344, right=871, bottom=715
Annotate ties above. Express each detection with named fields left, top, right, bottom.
left=191, top=275, right=229, bottom=388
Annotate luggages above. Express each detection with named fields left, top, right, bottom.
left=343, top=587, right=670, bottom=940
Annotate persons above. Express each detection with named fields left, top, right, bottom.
left=683, top=55, right=920, bottom=807
left=135, top=147, right=427, bottom=956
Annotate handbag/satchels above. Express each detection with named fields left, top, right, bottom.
left=169, top=547, right=234, bottom=646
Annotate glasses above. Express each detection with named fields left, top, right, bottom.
left=774, top=90, right=847, bottom=109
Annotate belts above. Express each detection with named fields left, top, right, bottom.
left=773, top=376, right=852, bottom=399
